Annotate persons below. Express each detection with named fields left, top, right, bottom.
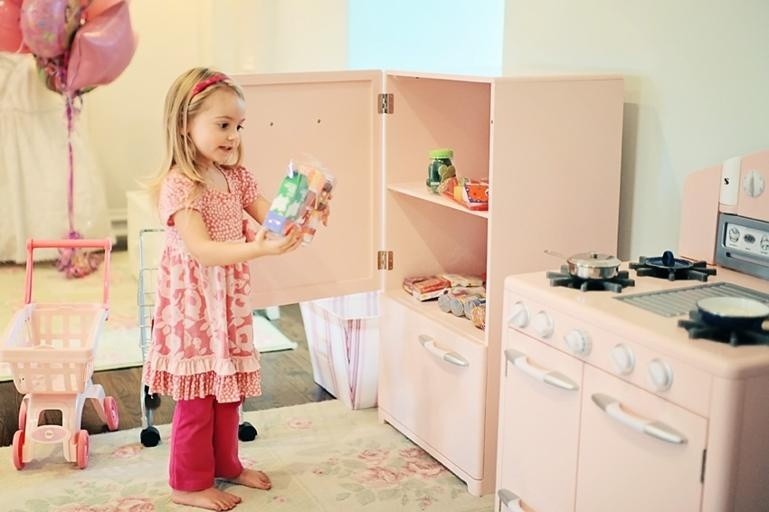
left=161, top=66, right=332, bottom=512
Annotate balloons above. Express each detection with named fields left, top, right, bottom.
left=79, top=2, right=120, bottom=24
left=63, top=1, right=137, bottom=101
left=0, top=0, right=28, bottom=54
left=22, top=0, right=81, bottom=59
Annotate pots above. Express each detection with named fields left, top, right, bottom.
left=567, top=252, right=624, bottom=281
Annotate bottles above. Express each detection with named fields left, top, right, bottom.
left=427, top=150, right=457, bottom=194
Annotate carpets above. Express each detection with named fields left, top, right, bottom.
left=0, top=401, right=500, bottom=510
left=1, top=250, right=294, bottom=380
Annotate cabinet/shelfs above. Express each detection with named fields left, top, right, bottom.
left=227, top=72, right=626, bottom=498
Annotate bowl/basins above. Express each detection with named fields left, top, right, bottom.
left=696, top=295, right=768, bottom=337
